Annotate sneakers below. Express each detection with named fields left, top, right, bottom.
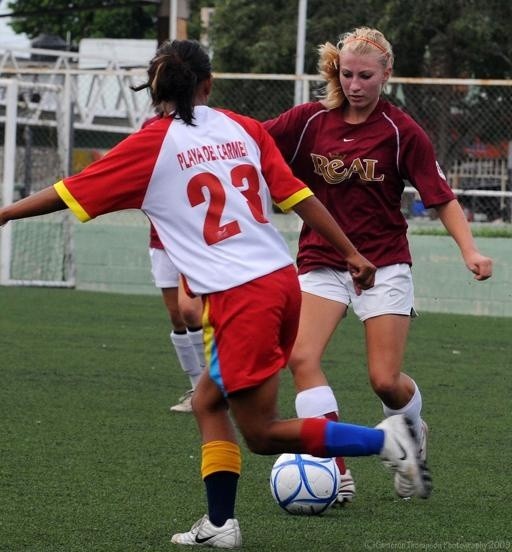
left=335, top=469, right=356, bottom=503
left=382, top=413, right=433, bottom=499
left=394, top=417, right=429, bottom=497
left=171, top=515, right=243, bottom=549
left=170, top=391, right=193, bottom=413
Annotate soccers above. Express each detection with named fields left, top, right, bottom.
left=270, top=451, right=340, bottom=517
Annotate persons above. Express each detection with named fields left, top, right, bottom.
left=0, top=28, right=493, bottom=546
